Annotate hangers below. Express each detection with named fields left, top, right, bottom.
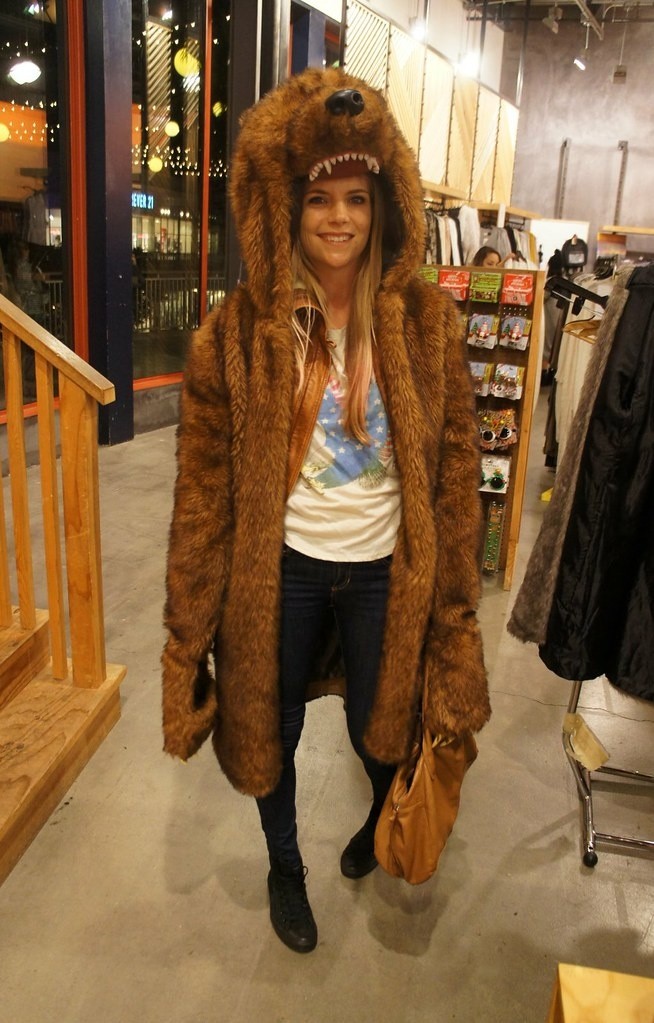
left=592, top=256, right=616, bottom=281
left=424, top=196, right=525, bottom=232
left=562, top=320, right=602, bottom=344
left=544, top=276, right=610, bottom=317
left=580, top=329, right=598, bottom=337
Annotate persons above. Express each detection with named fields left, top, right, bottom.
left=55, top=236, right=62, bottom=247
left=546, top=249, right=564, bottom=279
left=9, top=241, right=47, bottom=327
left=163, top=70, right=493, bottom=951
left=472, top=246, right=516, bottom=267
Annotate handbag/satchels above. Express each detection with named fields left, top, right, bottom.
left=373, top=724, right=478, bottom=884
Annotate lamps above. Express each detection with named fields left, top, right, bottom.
left=574, top=56, right=589, bottom=72
left=542, top=17, right=559, bottom=34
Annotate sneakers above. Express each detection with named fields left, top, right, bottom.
left=340, top=810, right=381, bottom=879
left=267, top=867, right=316, bottom=953
